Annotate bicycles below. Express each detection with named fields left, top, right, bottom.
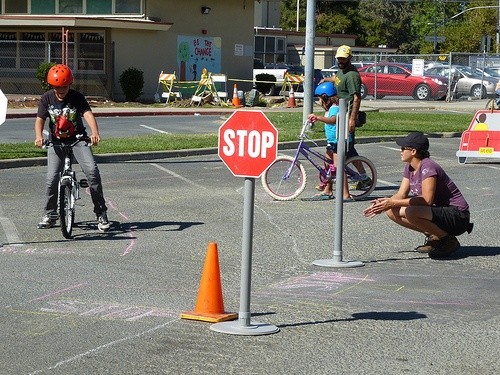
left=262, top=118, right=377, bottom=201
left=34, top=135, right=100, bottom=237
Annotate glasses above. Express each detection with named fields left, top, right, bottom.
left=400, top=146, right=414, bottom=152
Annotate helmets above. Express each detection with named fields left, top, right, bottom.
left=314, top=82, right=337, bottom=96
left=47, top=63, right=73, bottom=87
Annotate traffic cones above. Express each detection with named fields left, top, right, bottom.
left=285, top=87, right=298, bottom=107
left=180, top=243, right=239, bottom=323
left=230, top=84, right=244, bottom=108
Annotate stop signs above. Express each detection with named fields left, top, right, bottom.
left=217, top=109, right=279, bottom=178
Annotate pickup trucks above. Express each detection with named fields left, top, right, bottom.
left=253, top=63, right=325, bottom=96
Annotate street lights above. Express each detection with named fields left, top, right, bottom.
left=428, top=18, right=436, bottom=55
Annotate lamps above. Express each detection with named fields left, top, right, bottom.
left=201, top=7, right=211, bottom=14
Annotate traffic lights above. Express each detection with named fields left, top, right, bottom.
left=435, top=42, right=442, bottom=53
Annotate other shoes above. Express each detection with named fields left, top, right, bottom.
left=315, top=183, right=327, bottom=191
left=312, top=192, right=334, bottom=199
left=343, top=195, right=354, bottom=202
left=350, top=177, right=373, bottom=190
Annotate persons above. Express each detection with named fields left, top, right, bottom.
left=307, top=82, right=353, bottom=201
left=34, top=63, right=110, bottom=231
left=363, top=131, right=470, bottom=253
left=318, top=45, right=373, bottom=190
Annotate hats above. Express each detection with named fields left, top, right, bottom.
left=395, top=132, right=429, bottom=151
left=334, top=44, right=352, bottom=58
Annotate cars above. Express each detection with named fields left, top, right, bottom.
left=325, top=60, right=500, bottom=102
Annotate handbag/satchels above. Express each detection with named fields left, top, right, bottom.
left=355, top=110, right=366, bottom=128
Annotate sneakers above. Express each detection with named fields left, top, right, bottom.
left=37, top=215, right=56, bottom=229
left=428, top=236, right=461, bottom=259
left=96, top=210, right=111, bottom=233
left=417, top=233, right=439, bottom=253
